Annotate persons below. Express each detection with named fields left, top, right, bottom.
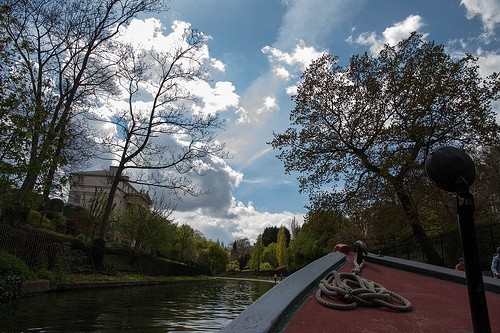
left=491, top=247, right=500, bottom=279
left=456, top=257, right=465, bottom=271
